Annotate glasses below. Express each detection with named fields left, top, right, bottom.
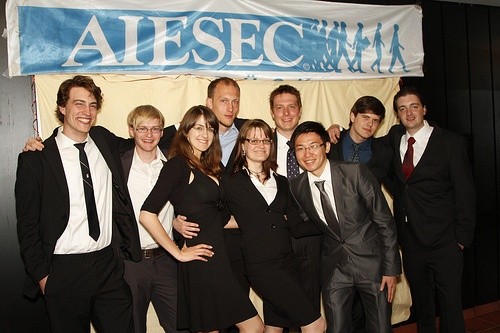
left=136, top=127, right=162, bottom=134
left=295, top=143, right=324, bottom=154
left=192, top=126, right=215, bottom=135
left=245, top=138, right=273, bottom=146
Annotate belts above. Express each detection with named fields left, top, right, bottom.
left=141, top=249, right=164, bottom=259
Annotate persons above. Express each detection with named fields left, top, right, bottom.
left=287, top=89, right=478, bottom=333
left=14, top=75, right=303, bottom=333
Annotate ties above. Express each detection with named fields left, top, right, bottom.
left=286, top=139, right=300, bottom=179
left=74, top=141, right=100, bottom=242
left=314, top=180, right=340, bottom=238
left=347, top=144, right=361, bottom=164
left=401, top=137, right=416, bottom=182
